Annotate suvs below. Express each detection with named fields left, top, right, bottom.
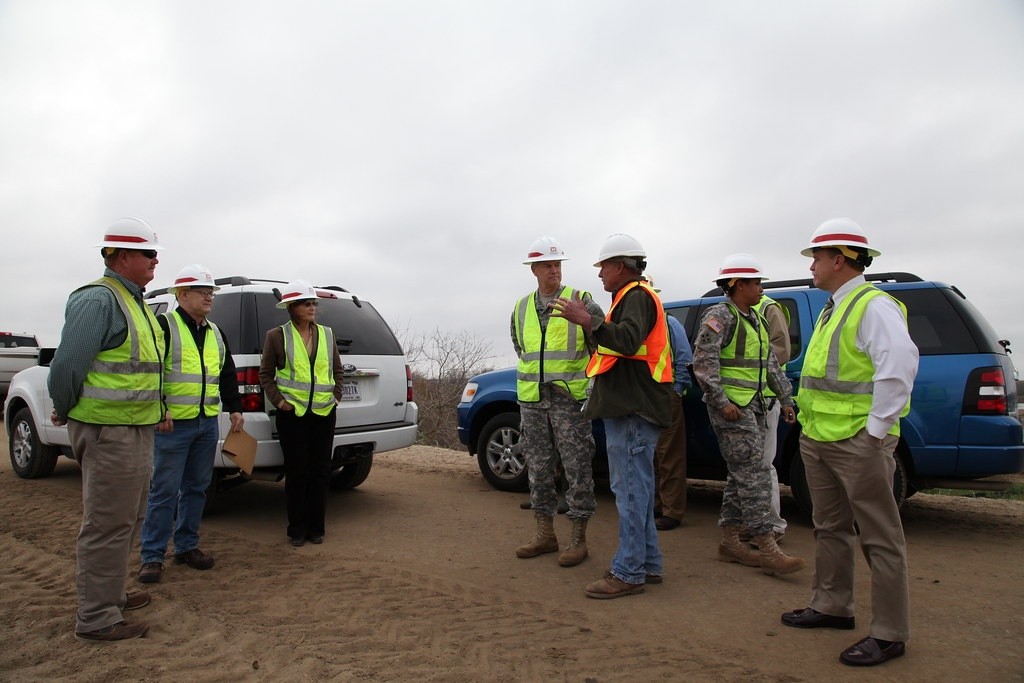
left=458, top=272, right=1024, bottom=523
left=4, top=276, right=418, bottom=487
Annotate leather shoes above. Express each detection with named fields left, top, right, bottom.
left=840, top=636, right=905, bottom=665
left=781, top=607, right=855, bottom=630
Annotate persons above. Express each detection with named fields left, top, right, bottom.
left=139, top=264, right=245, bottom=583
left=739, top=295, right=791, bottom=547
left=647, top=274, right=694, bottom=530
left=509, top=237, right=606, bottom=566
left=781, top=219, right=920, bottom=664
left=260, top=280, right=344, bottom=546
left=48, top=216, right=165, bottom=643
left=548, top=234, right=675, bottom=598
left=693, top=253, right=807, bottom=574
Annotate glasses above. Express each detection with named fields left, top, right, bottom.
left=191, top=290, right=216, bottom=300
left=305, top=301, right=316, bottom=307
left=142, top=250, right=157, bottom=259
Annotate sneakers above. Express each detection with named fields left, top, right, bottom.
left=646, top=575, right=664, bottom=584
left=123, top=590, right=152, bottom=610
left=585, top=574, right=645, bottom=598
left=310, top=536, right=323, bottom=544
left=292, top=537, right=304, bottom=546
left=138, top=562, right=165, bottom=583
left=74, top=621, right=149, bottom=642
left=175, top=548, right=214, bottom=571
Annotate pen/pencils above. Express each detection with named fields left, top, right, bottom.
left=221, top=449, right=237, bottom=456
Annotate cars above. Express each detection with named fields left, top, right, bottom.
left=0, top=331, right=42, bottom=395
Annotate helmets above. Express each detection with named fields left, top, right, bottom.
left=593, top=233, right=647, bottom=266
left=103, top=216, right=159, bottom=249
left=276, top=282, right=320, bottom=309
left=712, top=255, right=770, bottom=280
left=643, top=274, right=661, bottom=294
left=522, top=238, right=571, bottom=265
left=168, top=266, right=221, bottom=294
left=801, top=219, right=881, bottom=257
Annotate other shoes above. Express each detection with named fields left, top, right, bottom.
left=520, top=500, right=531, bottom=509
left=558, top=500, right=569, bottom=514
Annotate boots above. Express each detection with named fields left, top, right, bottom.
left=517, top=510, right=809, bottom=575
